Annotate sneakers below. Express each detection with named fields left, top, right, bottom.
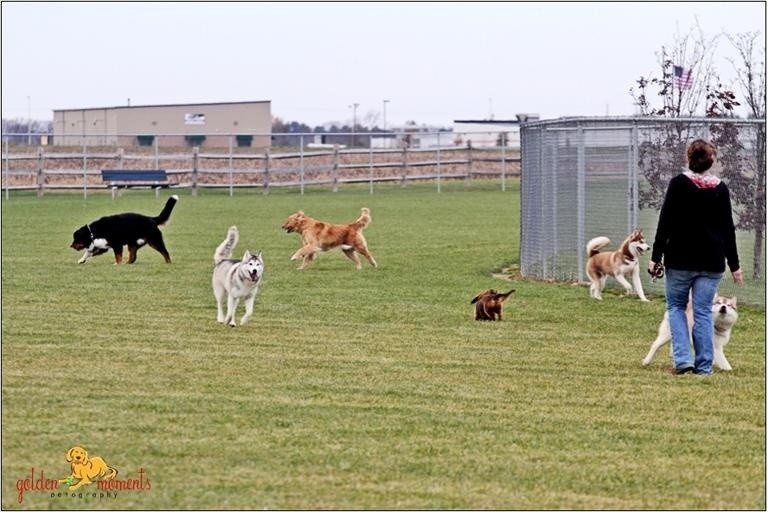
left=672, top=366, right=693, bottom=375
left=699, top=369, right=713, bottom=376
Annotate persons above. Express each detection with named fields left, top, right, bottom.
left=649, top=139, right=743, bottom=374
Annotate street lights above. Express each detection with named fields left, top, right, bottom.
left=382, top=100, right=391, bottom=144
left=229, top=122, right=238, bottom=197
left=349, top=104, right=357, bottom=146
left=92, top=109, right=107, bottom=144
left=72, top=111, right=88, bottom=199
left=150, top=121, right=160, bottom=197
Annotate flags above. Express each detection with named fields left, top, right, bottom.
left=672, top=65, right=693, bottom=89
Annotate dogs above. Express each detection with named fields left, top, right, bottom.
left=470, top=288, right=516, bottom=320
left=211, top=225, right=264, bottom=327
left=69, top=194, right=179, bottom=265
left=281, top=207, right=378, bottom=271
left=641, top=288, right=739, bottom=371
left=585, top=227, right=652, bottom=303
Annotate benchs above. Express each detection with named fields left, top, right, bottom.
left=102, top=170, right=180, bottom=200
